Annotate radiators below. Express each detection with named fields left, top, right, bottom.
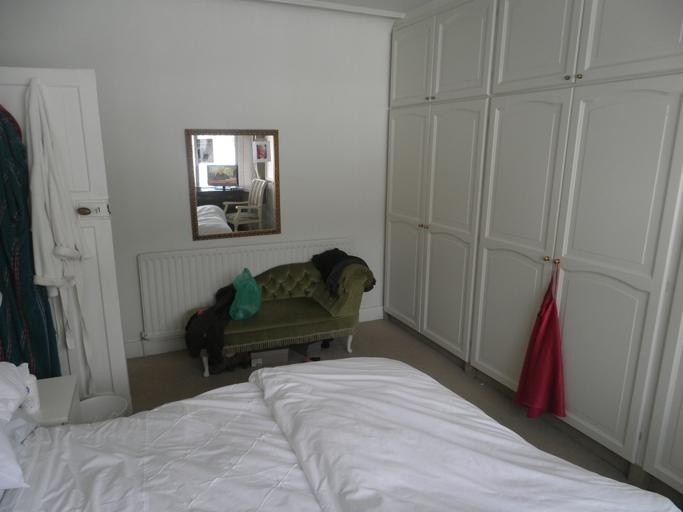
left=133, top=238, right=354, bottom=342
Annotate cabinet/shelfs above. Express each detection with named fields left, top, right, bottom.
left=469, top=5, right=679, bottom=480
left=385, top=1, right=489, bottom=370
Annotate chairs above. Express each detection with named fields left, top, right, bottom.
left=222, top=180, right=265, bottom=232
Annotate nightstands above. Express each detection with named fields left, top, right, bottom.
left=22, top=375, right=81, bottom=426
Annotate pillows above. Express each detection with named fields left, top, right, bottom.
left=0, top=362, right=39, bottom=494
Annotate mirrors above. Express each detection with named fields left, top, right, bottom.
left=180, top=127, right=285, bottom=242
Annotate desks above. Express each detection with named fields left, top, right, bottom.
left=197, top=187, right=241, bottom=211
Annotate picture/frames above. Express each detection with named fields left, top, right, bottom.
left=251, top=140, right=271, bottom=163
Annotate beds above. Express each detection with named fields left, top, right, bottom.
left=196, top=205, right=232, bottom=234
left=0, top=352, right=680, bottom=511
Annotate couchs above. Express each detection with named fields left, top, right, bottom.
left=194, top=252, right=370, bottom=379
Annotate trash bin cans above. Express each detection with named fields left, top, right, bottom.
left=68, top=396, right=128, bottom=424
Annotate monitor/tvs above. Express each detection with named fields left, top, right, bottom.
left=207, top=165, right=238, bottom=190
